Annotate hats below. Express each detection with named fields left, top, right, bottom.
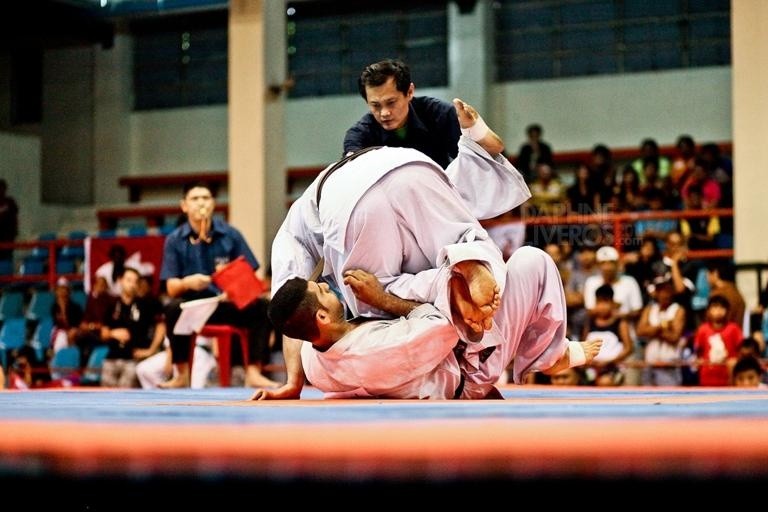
left=596, top=246, right=618, bottom=263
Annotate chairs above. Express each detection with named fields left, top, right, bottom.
left=1, top=215, right=183, bottom=387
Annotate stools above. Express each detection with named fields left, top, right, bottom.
left=183, top=324, right=252, bottom=389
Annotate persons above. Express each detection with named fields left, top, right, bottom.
left=1, top=57, right=767, bottom=402
